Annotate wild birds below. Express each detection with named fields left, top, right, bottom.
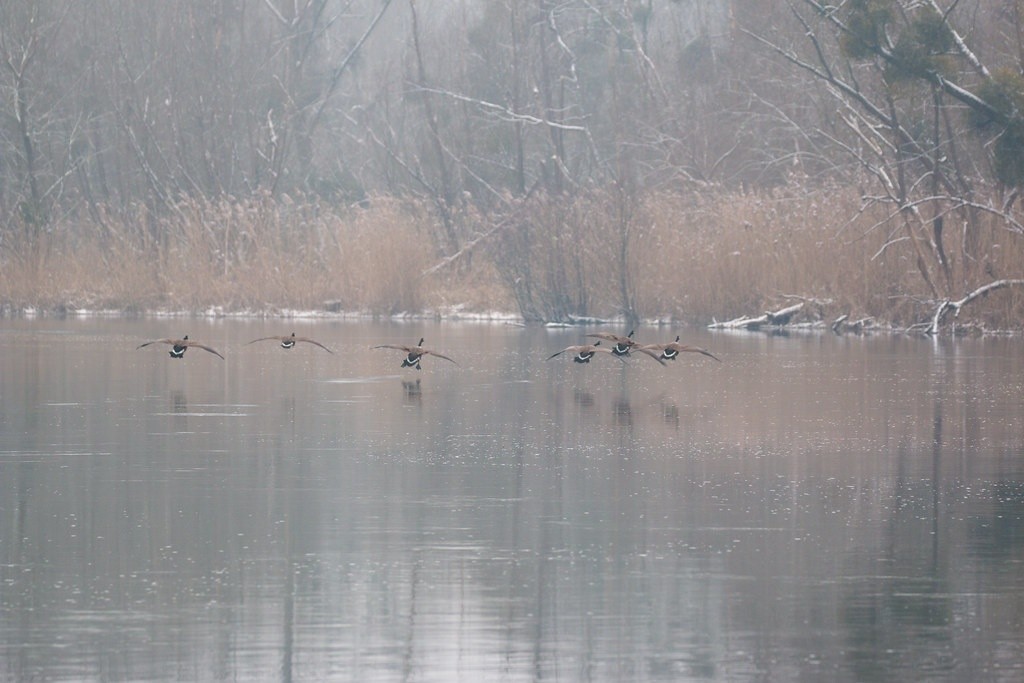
left=136, top=334, right=225, bottom=361
left=367, top=337, right=462, bottom=372
left=240, top=331, right=335, bottom=354
left=546, top=329, right=724, bottom=367
